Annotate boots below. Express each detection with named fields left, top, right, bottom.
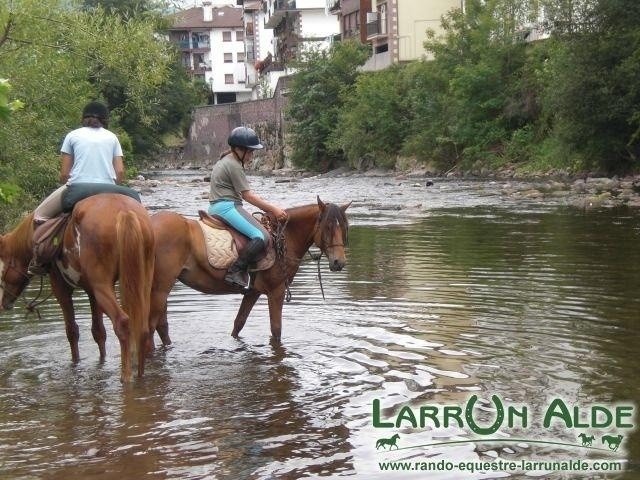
left=29, top=219, right=48, bottom=273
left=224, top=237, right=265, bottom=290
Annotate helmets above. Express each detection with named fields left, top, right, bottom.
left=228, top=127, right=264, bottom=150
left=84, top=102, right=108, bottom=119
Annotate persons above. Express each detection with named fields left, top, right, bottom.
left=208, top=126, right=289, bottom=287
left=27, top=101, right=124, bottom=277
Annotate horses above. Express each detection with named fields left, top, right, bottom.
left=145, top=195, right=354, bottom=357
left=0, top=192, right=157, bottom=384
